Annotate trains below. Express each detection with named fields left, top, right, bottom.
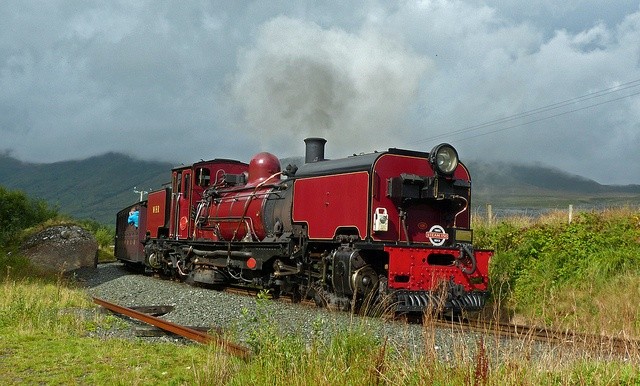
left=114, top=137, right=495, bottom=324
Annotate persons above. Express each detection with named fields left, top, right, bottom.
left=128, top=205, right=139, bottom=227
